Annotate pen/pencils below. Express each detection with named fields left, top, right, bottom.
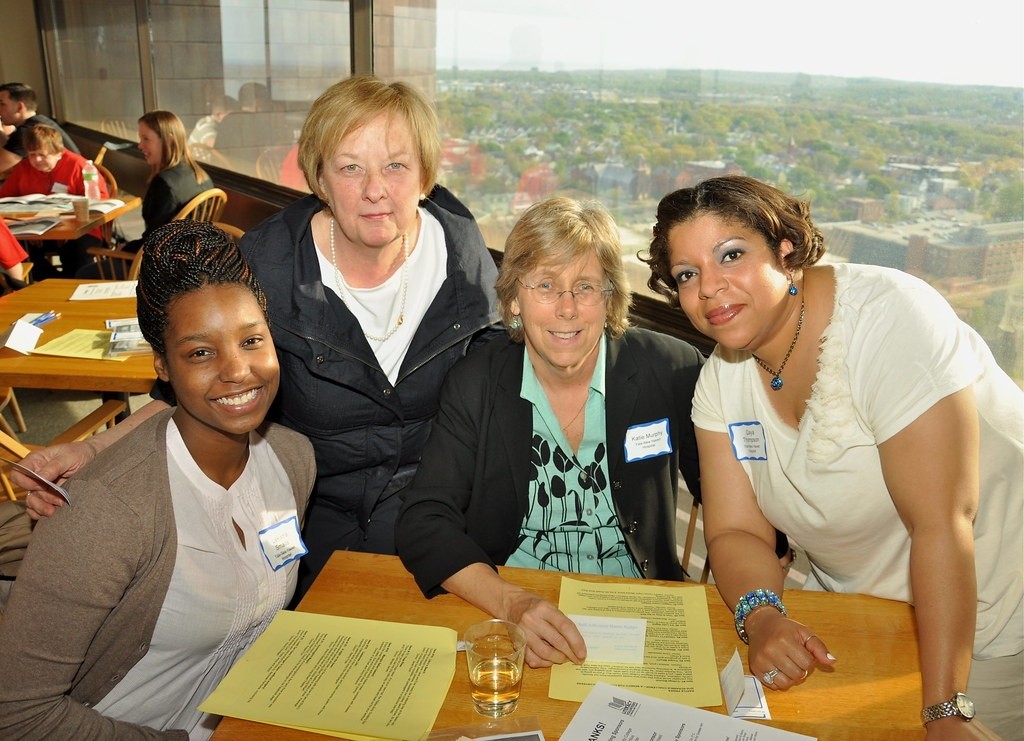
left=29, top=309, right=62, bottom=327
left=12, top=320, right=17, bottom=326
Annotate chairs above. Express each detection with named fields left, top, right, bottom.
left=0, top=386, right=128, bottom=506
left=92, top=163, right=117, bottom=199
left=87, top=188, right=230, bottom=283
left=128, top=221, right=246, bottom=281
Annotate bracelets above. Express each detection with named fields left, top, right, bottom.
left=733, top=589, right=788, bottom=644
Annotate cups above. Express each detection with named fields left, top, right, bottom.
left=72, top=198, right=89, bottom=222
left=463, top=619, right=527, bottom=720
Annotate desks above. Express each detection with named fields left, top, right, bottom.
left=0, top=197, right=142, bottom=279
left=208, top=548, right=929, bottom=741
left=0, top=279, right=175, bottom=422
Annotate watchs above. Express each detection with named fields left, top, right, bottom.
left=920, top=692, right=976, bottom=729
left=782, top=549, right=797, bottom=570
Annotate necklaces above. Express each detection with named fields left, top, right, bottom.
left=751, top=300, right=804, bottom=390
left=330, top=218, right=408, bottom=341
left=561, top=399, right=587, bottom=435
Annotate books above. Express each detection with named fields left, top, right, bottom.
left=0, top=193, right=125, bottom=237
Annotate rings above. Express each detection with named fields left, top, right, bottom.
left=763, top=668, right=779, bottom=684
left=27, top=490, right=32, bottom=496
left=803, top=634, right=816, bottom=646
left=801, top=670, right=808, bottom=679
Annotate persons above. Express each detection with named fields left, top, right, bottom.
left=186, top=83, right=311, bottom=193
left=395, top=197, right=796, bottom=669
left=637, top=175, right=1024, bottom=741
left=10, top=75, right=505, bottom=610
left=0, top=124, right=113, bottom=278
left=0, top=219, right=317, bottom=741
left=73, top=112, right=214, bottom=281
left=0, top=82, right=78, bottom=295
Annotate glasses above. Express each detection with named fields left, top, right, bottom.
left=516, top=277, right=614, bottom=307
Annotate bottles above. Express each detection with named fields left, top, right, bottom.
left=82, top=160, right=101, bottom=201
